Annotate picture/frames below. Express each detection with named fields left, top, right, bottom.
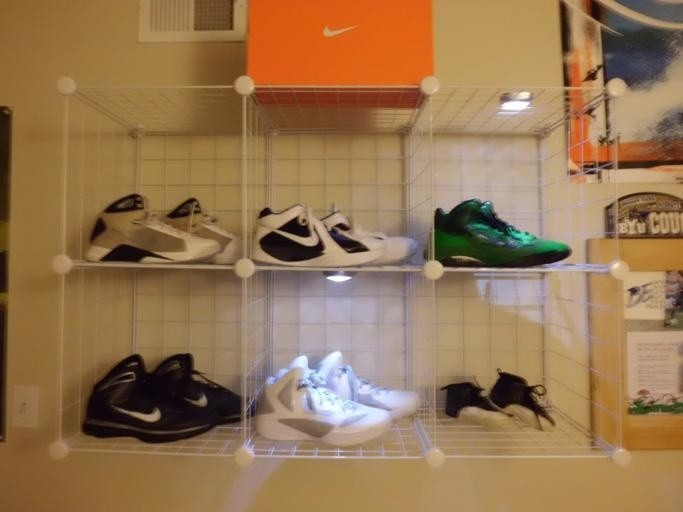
left=560, top=1, right=683, bottom=167
left=586, top=237, right=683, bottom=452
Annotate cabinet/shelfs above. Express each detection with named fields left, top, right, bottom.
left=49, top=76, right=632, bottom=468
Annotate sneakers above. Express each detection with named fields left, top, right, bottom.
left=255, top=351, right=420, bottom=447
left=251, top=203, right=419, bottom=268
left=85, top=194, right=241, bottom=265
left=428, top=198, right=573, bottom=267
left=82, top=353, right=258, bottom=443
left=440, top=368, right=556, bottom=430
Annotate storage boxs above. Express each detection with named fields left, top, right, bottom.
left=246, top=2, right=433, bottom=111
